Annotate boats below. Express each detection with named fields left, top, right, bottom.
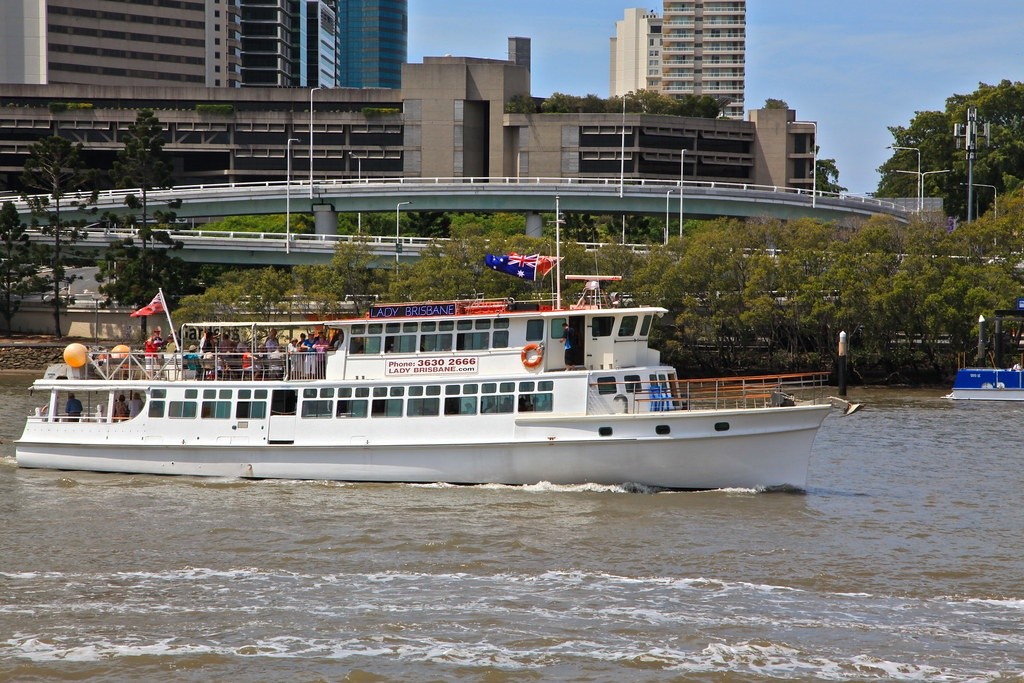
left=19, top=196, right=867, bottom=504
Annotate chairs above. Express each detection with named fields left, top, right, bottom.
left=182, top=352, right=286, bottom=381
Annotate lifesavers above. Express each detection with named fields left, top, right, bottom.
left=521, top=343, right=542, bottom=368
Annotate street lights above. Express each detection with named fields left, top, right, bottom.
left=681, top=148, right=688, bottom=238
left=788, top=118, right=816, bottom=209
left=664, top=189, right=675, bottom=247
left=286, top=138, right=303, bottom=255
left=958, top=179, right=997, bottom=253
left=884, top=144, right=949, bottom=214
left=396, top=201, right=415, bottom=274
left=309, top=88, right=324, bottom=200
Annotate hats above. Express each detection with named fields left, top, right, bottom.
left=134, top=393, right=140, bottom=399
left=69, top=393, right=74, bottom=396
left=189, top=345, right=197, bottom=351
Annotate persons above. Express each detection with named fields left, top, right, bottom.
left=144, top=326, right=361, bottom=382
left=559, top=323, right=575, bottom=372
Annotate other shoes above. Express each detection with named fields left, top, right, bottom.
left=196, top=374, right=284, bottom=381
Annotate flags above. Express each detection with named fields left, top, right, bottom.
left=131, top=293, right=164, bottom=317
left=486, top=252, right=563, bottom=282
